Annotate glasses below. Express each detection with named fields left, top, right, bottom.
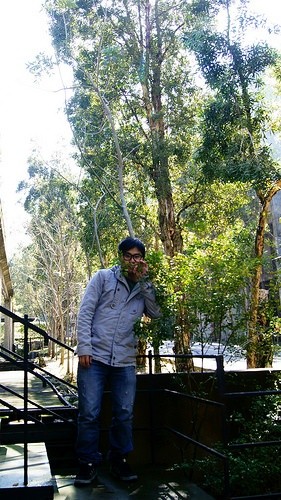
left=123, top=251, right=142, bottom=263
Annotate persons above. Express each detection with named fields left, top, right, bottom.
left=73, top=237, right=162, bottom=488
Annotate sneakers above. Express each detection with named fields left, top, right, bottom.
left=111, top=457, right=138, bottom=480
left=74, top=464, right=97, bottom=484
